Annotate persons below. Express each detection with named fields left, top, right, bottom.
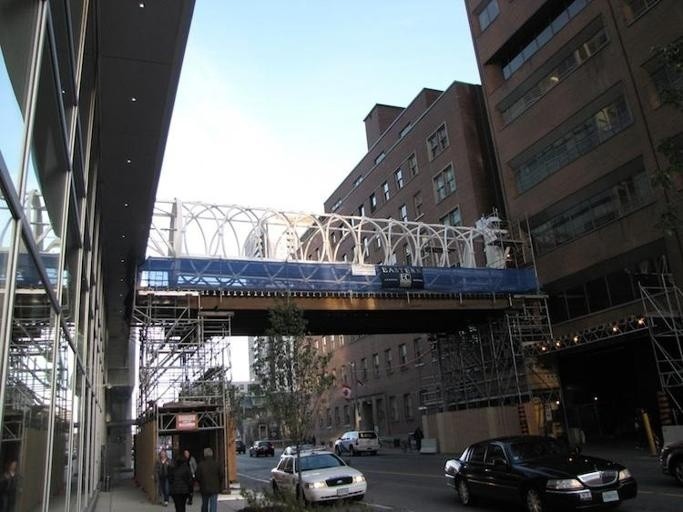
left=184, top=450, right=199, bottom=504
left=196, top=448, right=227, bottom=512
left=151, top=448, right=172, bottom=506
left=169, top=453, right=194, bottom=511
left=1, top=460, right=23, bottom=510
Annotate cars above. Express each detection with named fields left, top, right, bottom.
left=280, top=444, right=317, bottom=463
left=443, top=435, right=637, bottom=512
left=235, top=441, right=245, bottom=454
left=248, top=441, right=274, bottom=457
left=658, top=439, right=682, bottom=486
left=270, top=451, right=366, bottom=508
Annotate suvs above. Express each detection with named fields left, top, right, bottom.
left=333, top=430, right=380, bottom=458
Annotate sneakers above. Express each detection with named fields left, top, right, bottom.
left=163, top=500, right=193, bottom=506
